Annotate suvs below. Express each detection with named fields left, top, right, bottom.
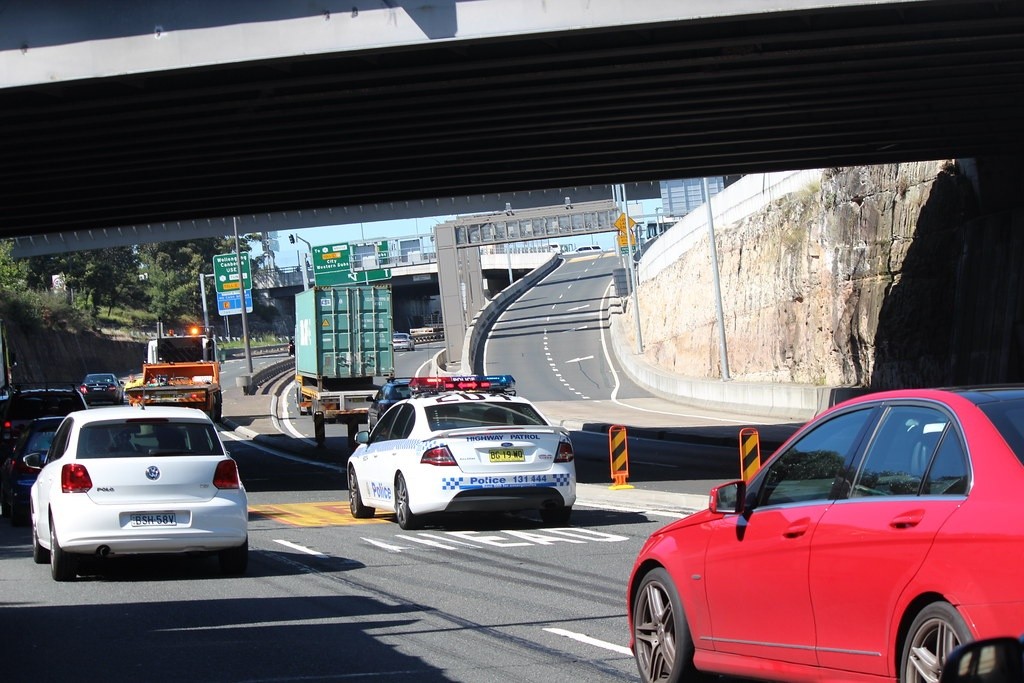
left=567, top=246, right=602, bottom=255
left=544, top=244, right=561, bottom=255
left=3, top=381, right=92, bottom=448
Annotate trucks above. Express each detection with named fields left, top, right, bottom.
left=128, top=324, right=222, bottom=422
left=292, top=286, right=396, bottom=423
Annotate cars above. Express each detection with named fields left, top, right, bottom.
left=625, top=384, right=1024, bottom=683
left=79, top=374, right=126, bottom=404
left=391, top=332, right=416, bottom=351
left=23, top=406, right=249, bottom=580
left=363, top=375, right=417, bottom=428
left=3, top=417, right=70, bottom=526
left=347, top=374, right=579, bottom=530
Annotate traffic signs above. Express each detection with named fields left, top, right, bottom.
left=311, top=238, right=390, bottom=288
left=217, top=289, right=253, bottom=315
left=213, top=253, right=251, bottom=292
